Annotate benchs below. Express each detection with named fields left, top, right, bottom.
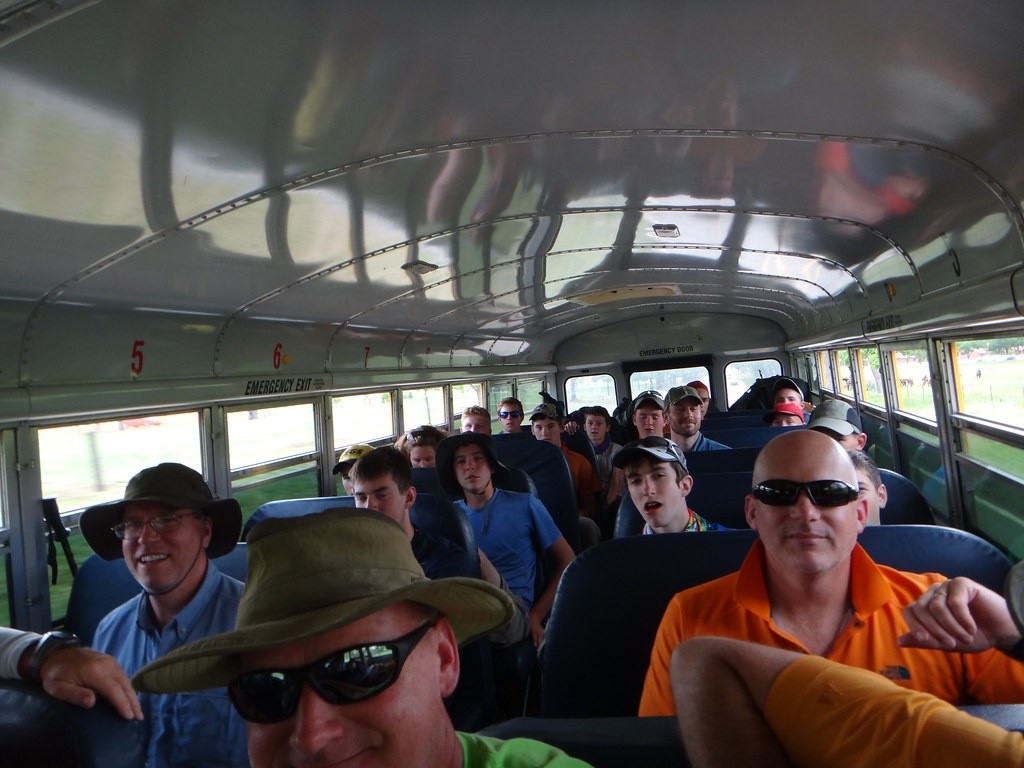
left=0, top=379, right=1024, bottom=767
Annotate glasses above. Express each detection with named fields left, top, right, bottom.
left=110, top=511, right=196, bottom=539
left=227, top=614, right=440, bottom=723
left=498, top=410, right=521, bottom=419
left=750, top=479, right=858, bottom=506
left=633, top=391, right=662, bottom=406
left=622, top=436, right=689, bottom=472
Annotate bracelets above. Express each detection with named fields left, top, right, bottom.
left=1007, top=638, right=1024, bottom=663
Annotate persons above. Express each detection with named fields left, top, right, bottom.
left=564, top=406, right=626, bottom=516
left=632, top=390, right=665, bottom=439
left=333, top=444, right=374, bottom=497
left=772, top=378, right=805, bottom=410
left=636, top=429, right=1024, bottom=717
left=662, top=386, right=732, bottom=451
left=460, top=405, right=492, bottom=434
left=671, top=635, right=1024, bottom=768
left=846, top=446, right=887, bottom=526
left=529, top=403, right=602, bottom=549
left=0, top=626, right=144, bottom=721
left=129, top=506, right=593, bottom=768
left=349, top=446, right=479, bottom=580
left=611, top=435, right=735, bottom=534
left=79, top=462, right=247, bottom=768
left=687, top=381, right=710, bottom=420
left=762, top=403, right=807, bottom=427
left=397, top=424, right=446, bottom=469
left=434, top=430, right=575, bottom=647
left=805, top=399, right=867, bottom=452
left=497, top=397, right=525, bottom=434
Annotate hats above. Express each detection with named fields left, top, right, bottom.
left=435, top=433, right=512, bottom=493
left=687, top=381, right=709, bottom=400
left=130, top=507, right=516, bottom=694
left=529, top=404, right=559, bottom=422
left=79, top=462, right=242, bottom=560
left=612, top=437, right=689, bottom=471
left=662, top=386, right=702, bottom=412
left=806, top=398, right=862, bottom=436
left=333, top=444, right=375, bottom=475
left=772, top=402, right=804, bottom=423
left=769, top=377, right=805, bottom=403
left=633, top=392, right=665, bottom=413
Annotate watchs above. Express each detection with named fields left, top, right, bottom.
left=30, top=630, right=79, bottom=685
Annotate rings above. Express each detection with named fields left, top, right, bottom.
left=934, top=591, right=947, bottom=598
